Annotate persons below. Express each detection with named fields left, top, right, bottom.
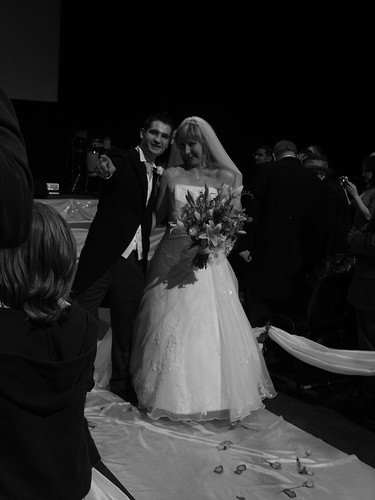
left=0, top=201, right=99, bottom=500
left=130, top=116, right=278, bottom=425
left=0, top=81, right=34, bottom=248
left=69, top=114, right=173, bottom=404
left=226, top=140, right=375, bottom=389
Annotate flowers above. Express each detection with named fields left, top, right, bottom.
left=168, top=183, right=253, bottom=269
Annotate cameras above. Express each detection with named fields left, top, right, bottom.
left=338, top=176, right=348, bottom=188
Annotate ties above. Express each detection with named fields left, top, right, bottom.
left=144, top=162, right=154, bottom=177
